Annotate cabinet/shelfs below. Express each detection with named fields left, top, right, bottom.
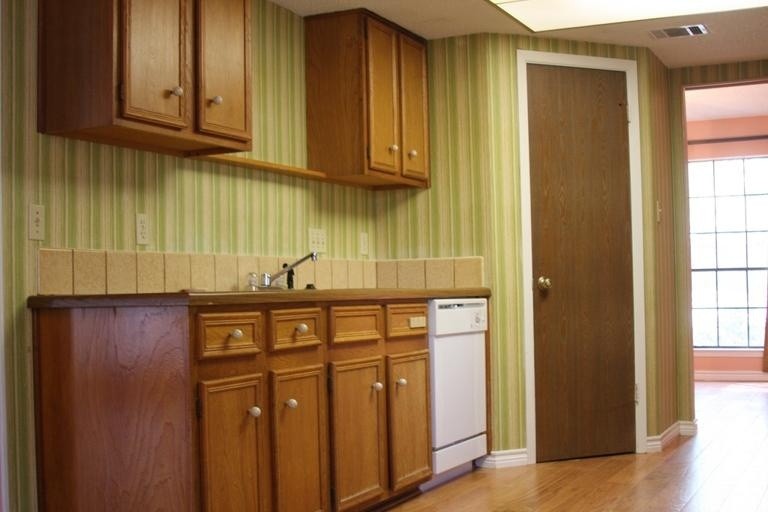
left=325, top=288, right=432, bottom=512
left=303, top=8, right=431, bottom=189
left=188, top=290, right=330, bottom=512
left=37, top=0, right=253, bottom=155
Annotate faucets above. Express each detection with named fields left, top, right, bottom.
left=259, top=251, right=318, bottom=288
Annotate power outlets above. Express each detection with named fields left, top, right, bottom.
left=28, top=202, right=45, bottom=241
left=136, top=213, right=149, bottom=246
